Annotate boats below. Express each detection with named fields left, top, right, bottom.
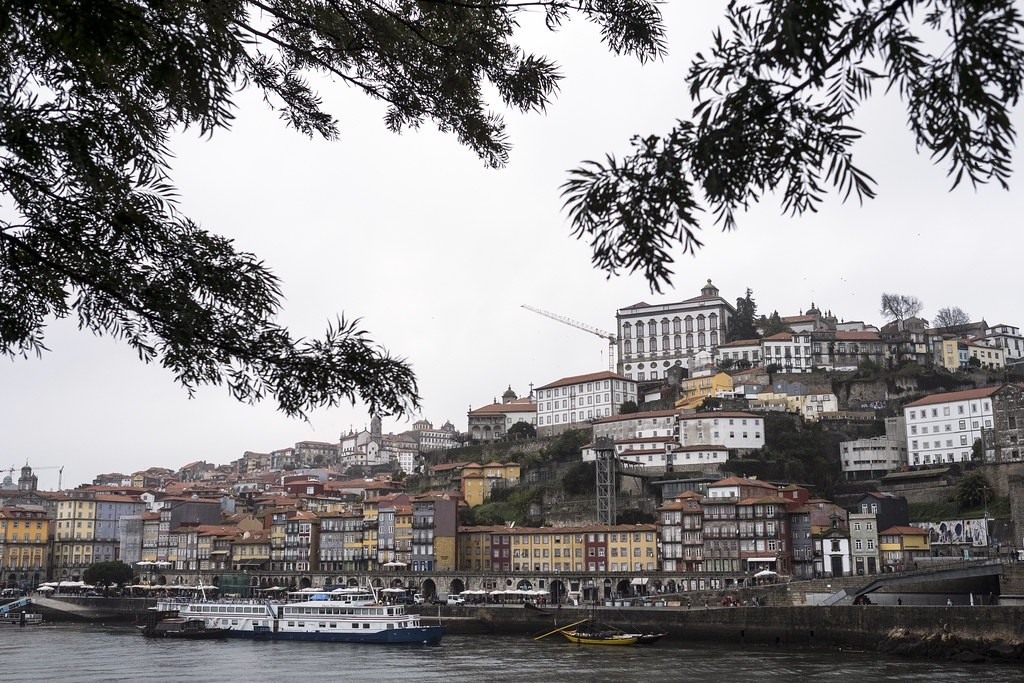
left=554, top=588, right=671, bottom=645
left=146, top=580, right=448, bottom=645
left=136, top=597, right=232, bottom=640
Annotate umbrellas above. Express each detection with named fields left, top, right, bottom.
left=34, top=557, right=552, bottom=606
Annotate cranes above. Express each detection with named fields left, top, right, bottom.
left=0, top=463, right=61, bottom=483
left=521, top=304, right=618, bottom=374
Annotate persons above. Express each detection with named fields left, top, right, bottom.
left=381, top=543, right=1017, bottom=608
